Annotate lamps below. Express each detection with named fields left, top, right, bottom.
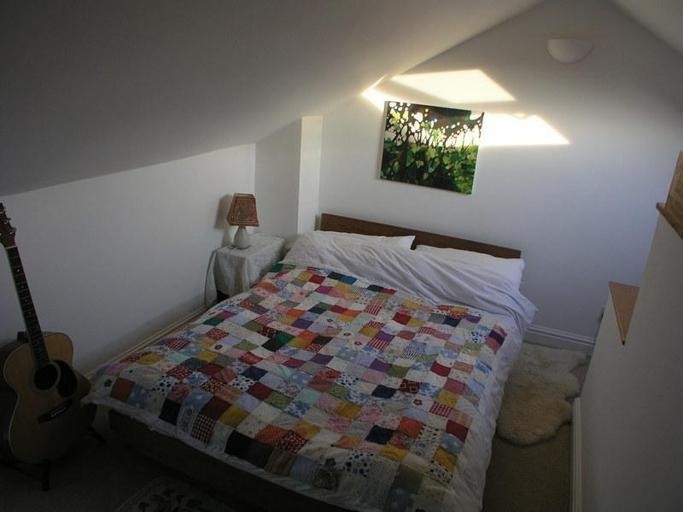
left=226, top=192, right=260, bottom=249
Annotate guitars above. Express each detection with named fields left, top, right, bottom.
left=1, top=204, right=92, bottom=465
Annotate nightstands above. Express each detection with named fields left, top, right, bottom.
left=215, top=233, right=284, bottom=300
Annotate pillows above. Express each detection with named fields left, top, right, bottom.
left=312, top=229, right=415, bottom=251
left=416, top=244, right=526, bottom=291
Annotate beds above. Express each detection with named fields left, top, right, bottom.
left=109, top=214, right=521, bottom=512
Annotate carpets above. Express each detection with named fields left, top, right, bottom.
left=114, top=473, right=242, bottom=512
left=496, top=341, right=587, bottom=446
